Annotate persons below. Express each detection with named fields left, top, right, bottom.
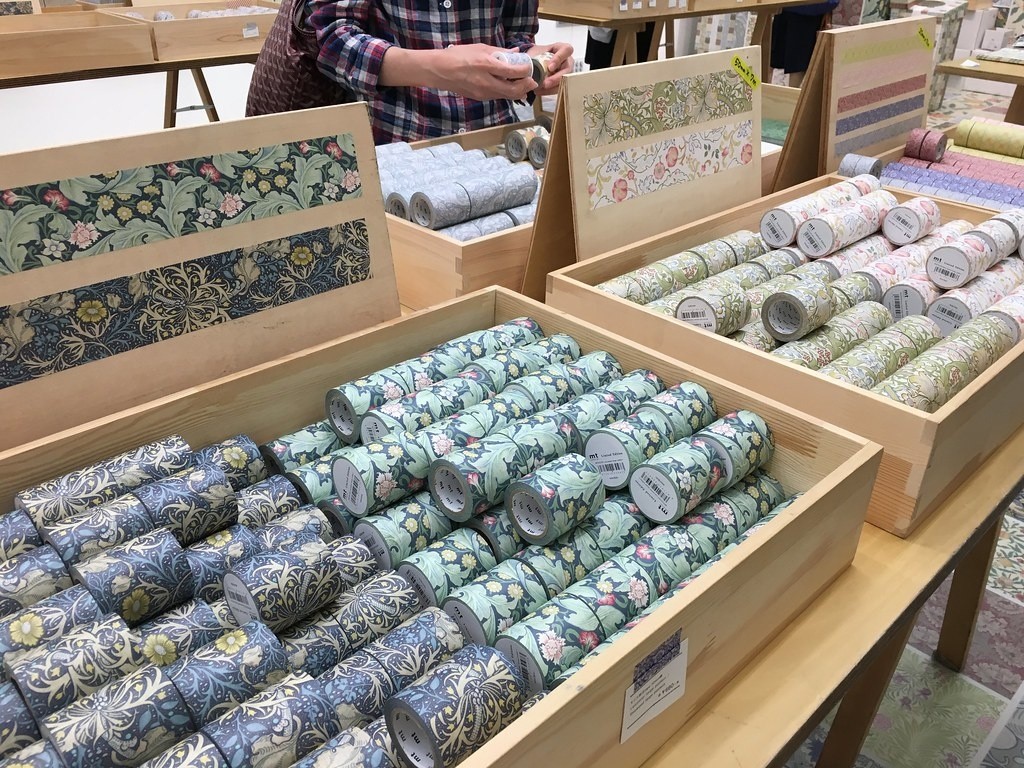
left=306, top=0, right=574, bottom=147
left=768, top=0, right=841, bottom=90
left=582, top=22, right=655, bottom=70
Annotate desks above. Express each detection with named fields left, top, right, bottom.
left=532, top=0, right=828, bottom=118
left=0, top=53, right=261, bottom=130
left=936, top=57, right=1024, bottom=126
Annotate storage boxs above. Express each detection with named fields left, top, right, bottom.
left=831, top=0, right=891, bottom=25
left=385, top=117, right=547, bottom=312
left=980, top=27, right=1014, bottom=51
left=889, top=0, right=923, bottom=10
left=946, top=49, right=972, bottom=88
left=830, top=123, right=1003, bottom=213
left=961, top=48, right=1024, bottom=97
left=760, top=82, right=801, bottom=195
left=545, top=173, right=1024, bottom=539
left=957, top=7, right=999, bottom=50
left=888, top=0, right=969, bottom=112
left=0, top=0, right=281, bottom=80
left=0, top=285, right=888, bottom=768
